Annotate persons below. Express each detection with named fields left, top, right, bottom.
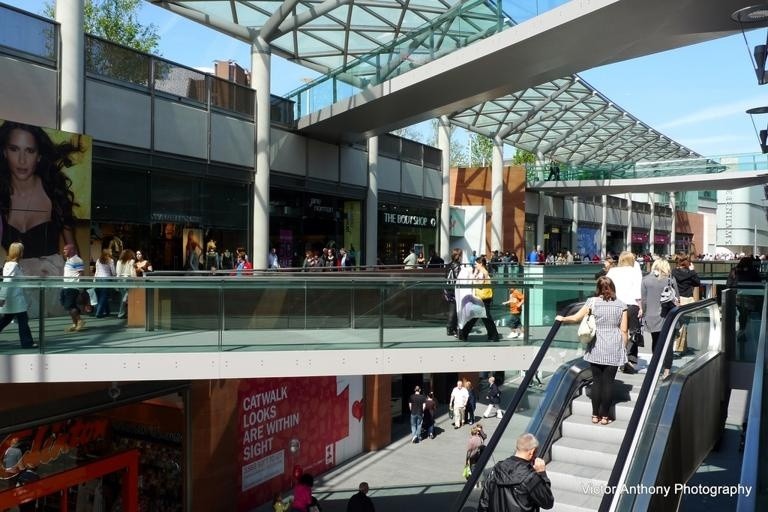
left=579, top=233, right=596, bottom=256
left=0, top=121, right=85, bottom=320
left=1, top=240, right=768, bottom=510
left=183, top=231, right=204, bottom=271
left=548, top=159, right=559, bottom=182
left=161, top=223, right=182, bottom=271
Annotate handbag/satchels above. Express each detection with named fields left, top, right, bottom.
left=443, top=289, right=455, bottom=302
left=660, top=285, right=677, bottom=309
left=630, top=332, right=645, bottom=348
left=86, top=288, right=98, bottom=305
left=578, top=314, right=596, bottom=344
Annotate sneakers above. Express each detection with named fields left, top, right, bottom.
left=454, top=420, right=473, bottom=429
left=67, top=320, right=86, bottom=331
left=23, top=342, right=37, bottom=348
left=507, top=332, right=525, bottom=340
left=412, top=433, right=434, bottom=443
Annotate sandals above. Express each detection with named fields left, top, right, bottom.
left=593, top=417, right=609, bottom=424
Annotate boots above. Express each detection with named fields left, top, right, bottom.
left=447, top=326, right=457, bottom=334
